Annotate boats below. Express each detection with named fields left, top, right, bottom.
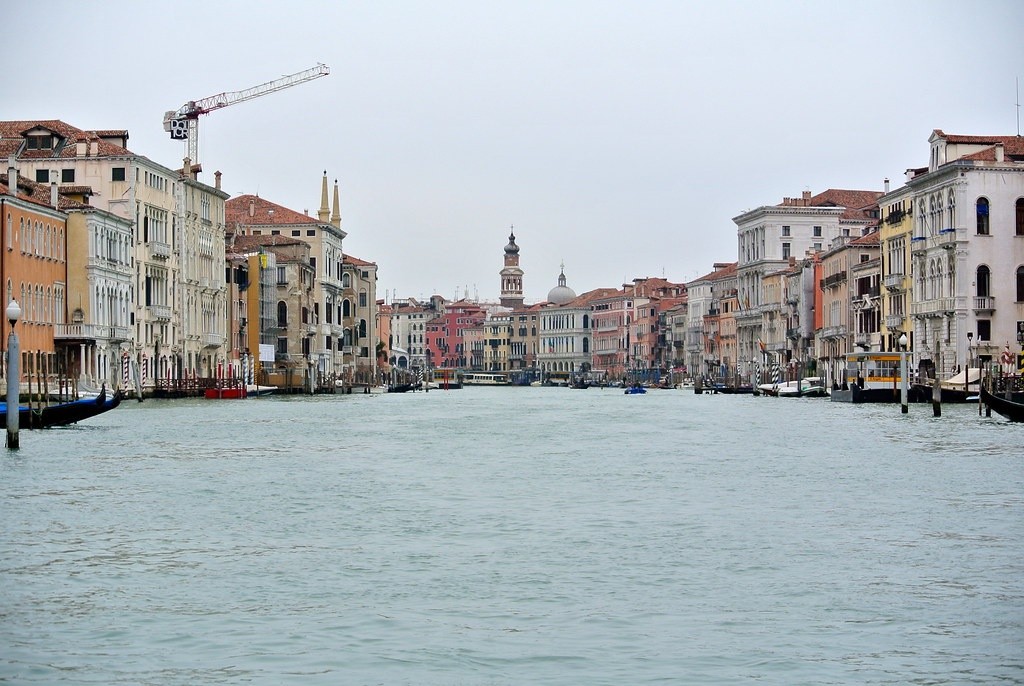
left=0, top=390, right=121, bottom=427
left=246, top=384, right=281, bottom=395
left=404, top=367, right=1023, bottom=426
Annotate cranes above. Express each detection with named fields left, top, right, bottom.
left=158, top=62, right=333, bottom=183
